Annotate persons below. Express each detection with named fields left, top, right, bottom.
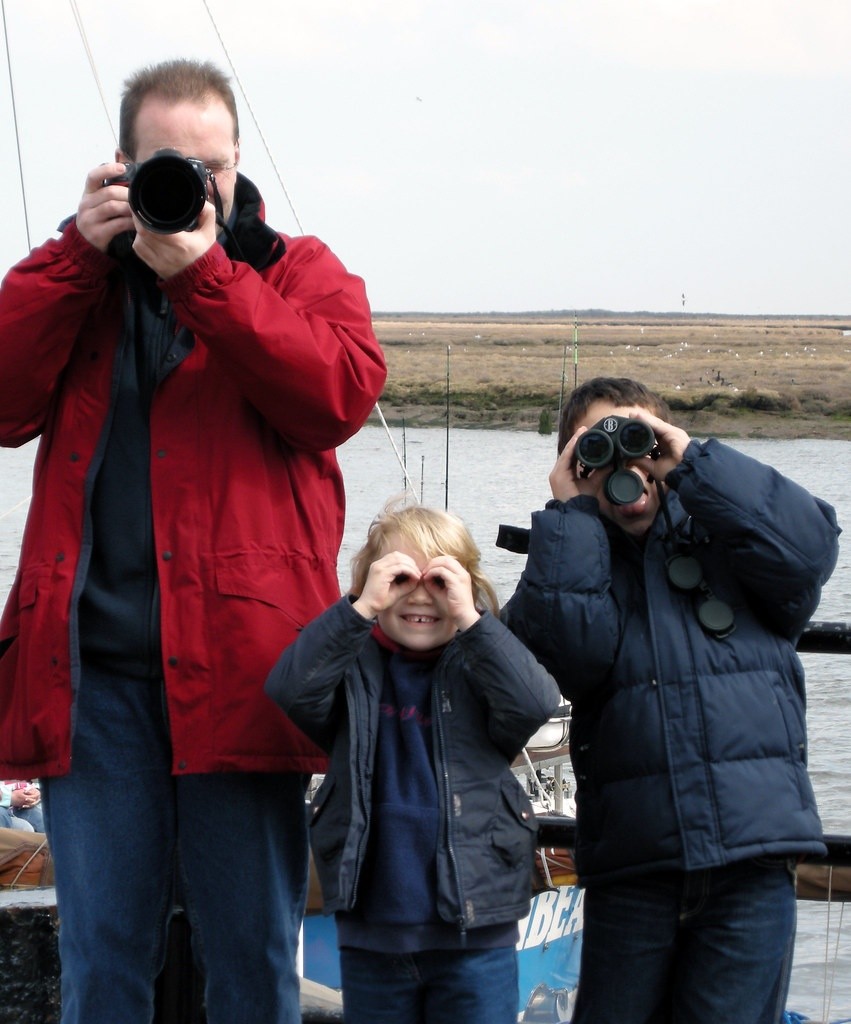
left=0, top=780, right=45, bottom=833
left=0, top=61, right=388, bottom=1024
left=497, top=378, right=841, bottom=1024
left=263, top=505, right=560, bottom=1024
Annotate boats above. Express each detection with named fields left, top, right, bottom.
left=2, top=3, right=851, bottom=1024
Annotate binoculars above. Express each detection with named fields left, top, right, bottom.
left=575, top=414, right=655, bottom=506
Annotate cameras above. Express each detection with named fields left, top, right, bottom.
left=104, top=147, right=208, bottom=234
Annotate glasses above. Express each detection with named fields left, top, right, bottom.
left=206, top=160, right=237, bottom=177
